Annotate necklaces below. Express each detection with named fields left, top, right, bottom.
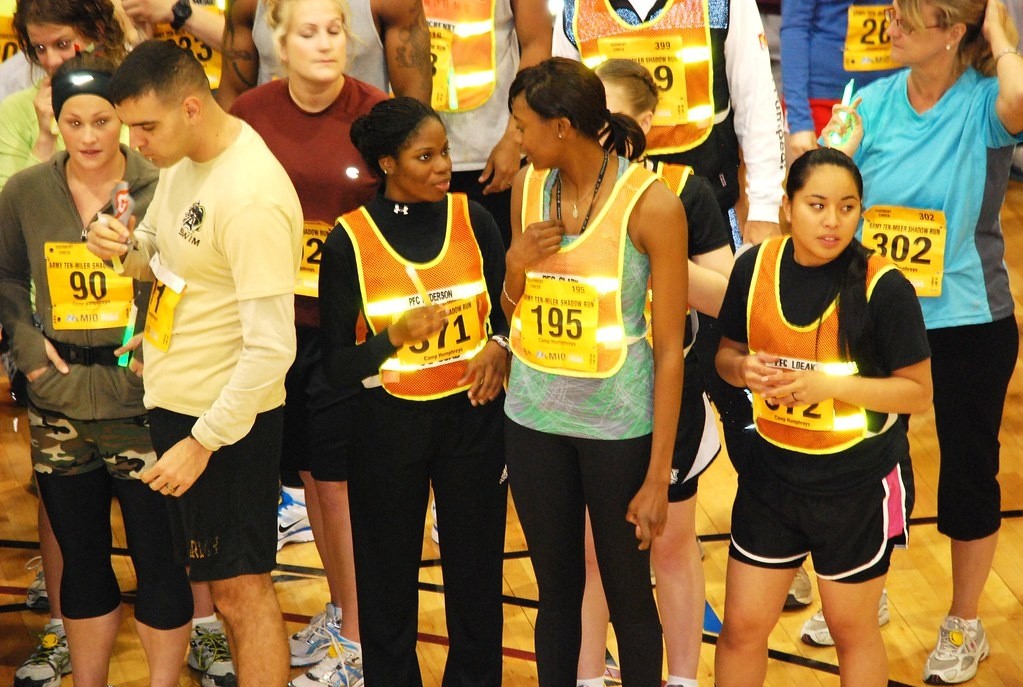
left=557, top=154, right=609, bottom=218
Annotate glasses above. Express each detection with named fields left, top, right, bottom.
left=884, top=8, right=952, bottom=35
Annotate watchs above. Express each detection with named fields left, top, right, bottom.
left=170, top=0, right=192, bottom=30
left=486, top=334, right=512, bottom=365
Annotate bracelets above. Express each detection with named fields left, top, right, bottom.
left=994, top=48, right=1023, bottom=71
left=503, top=281, right=516, bottom=305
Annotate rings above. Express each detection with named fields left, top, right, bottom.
left=792, top=392, right=798, bottom=402
left=166, top=484, right=175, bottom=492
left=508, top=182, right=512, bottom=186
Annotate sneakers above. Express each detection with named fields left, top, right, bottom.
left=14, top=624, right=72, bottom=686
left=924, top=615, right=989, bottom=683
left=783, top=565, right=812, bottom=607
left=277, top=492, right=315, bottom=550
left=801, top=588, right=890, bottom=645
left=187, top=624, right=236, bottom=687
left=286, top=625, right=362, bottom=687
left=23, top=556, right=49, bottom=611
left=286, top=604, right=344, bottom=665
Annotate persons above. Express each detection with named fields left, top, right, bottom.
left=0, top=1, right=1023, bottom=687
left=87, top=41, right=304, bottom=687
left=502, top=56, right=688, bottom=687
left=315, top=94, right=513, bottom=687
left=0, top=53, right=195, bottom=687
left=714, top=146, right=932, bottom=687
left=577, top=59, right=733, bottom=687
